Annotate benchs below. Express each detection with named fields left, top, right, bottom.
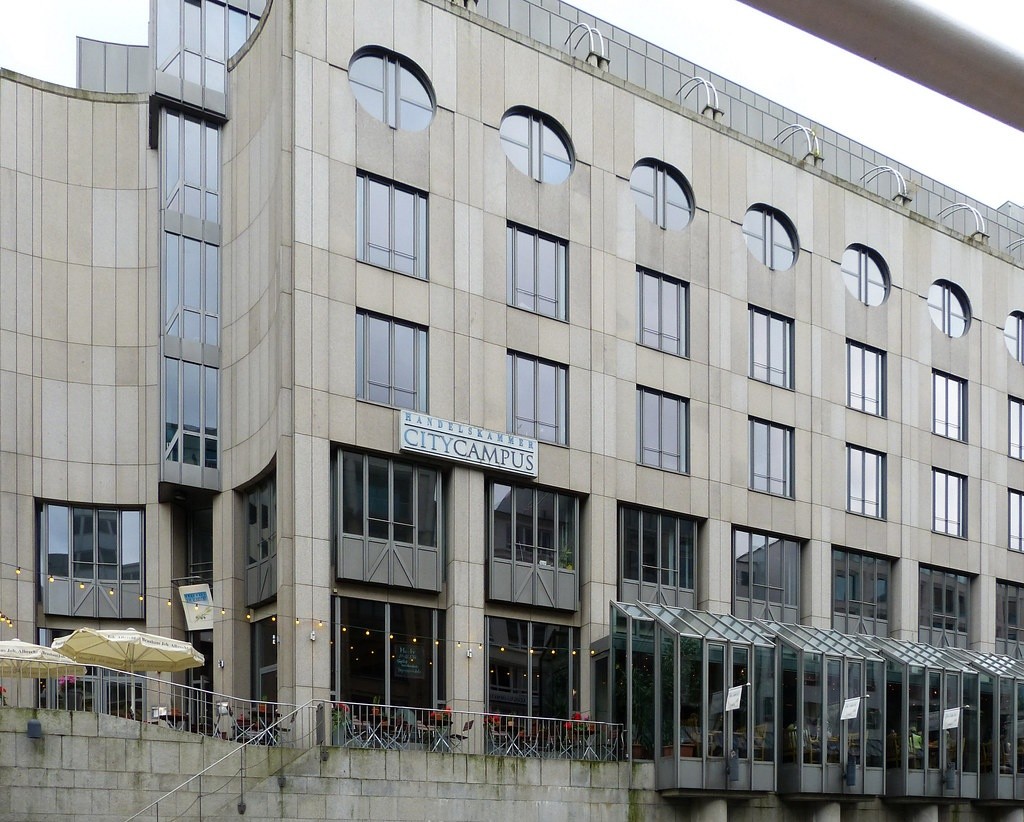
left=735, top=723, right=767, bottom=760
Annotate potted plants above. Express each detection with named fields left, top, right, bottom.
left=369, top=695, right=382, bottom=724
left=622, top=637, right=702, bottom=759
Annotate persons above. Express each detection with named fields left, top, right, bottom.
left=1006, top=741, right=1024, bottom=772
left=909, top=725, right=923, bottom=762
left=685, top=713, right=702, bottom=756
left=791, top=721, right=808, bottom=750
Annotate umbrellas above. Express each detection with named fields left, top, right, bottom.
left=0, top=627, right=205, bottom=721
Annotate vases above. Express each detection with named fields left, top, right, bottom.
left=333, top=725, right=344, bottom=746
left=63, top=692, right=83, bottom=711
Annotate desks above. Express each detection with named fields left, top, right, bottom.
left=421, top=720, right=453, bottom=753
left=159, top=715, right=188, bottom=729
left=494, top=726, right=524, bottom=756
left=248, top=711, right=282, bottom=746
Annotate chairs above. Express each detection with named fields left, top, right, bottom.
left=787, top=725, right=822, bottom=764
left=342, top=710, right=414, bottom=750
left=445, top=720, right=474, bottom=751
left=482, top=723, right=618, bottom=761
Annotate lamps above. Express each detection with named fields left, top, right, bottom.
left=941, top=762, right=955, bottom=789
left=842, top=756, right=856, bottom=786
left=27, top=719, right=42, bottom=739
left=218, top=660, right=224, bottom=668
left=726, top=749, right=739, bottom=782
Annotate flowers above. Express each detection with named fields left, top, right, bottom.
left=430, top=707, right=452, bottom=719
left=331, top=704, right=350, bottom=732
left=59, top=676, right=81, bottom=690
left=484, top=712, right=500, bottom=730
left=564, top=712, right=589, bottom=731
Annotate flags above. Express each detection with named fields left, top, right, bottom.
left=840, top=697, right=860, bottom=720
left=725, top=687, right=742, bottom=711
left=942, top=707, right=960, bottom=730
